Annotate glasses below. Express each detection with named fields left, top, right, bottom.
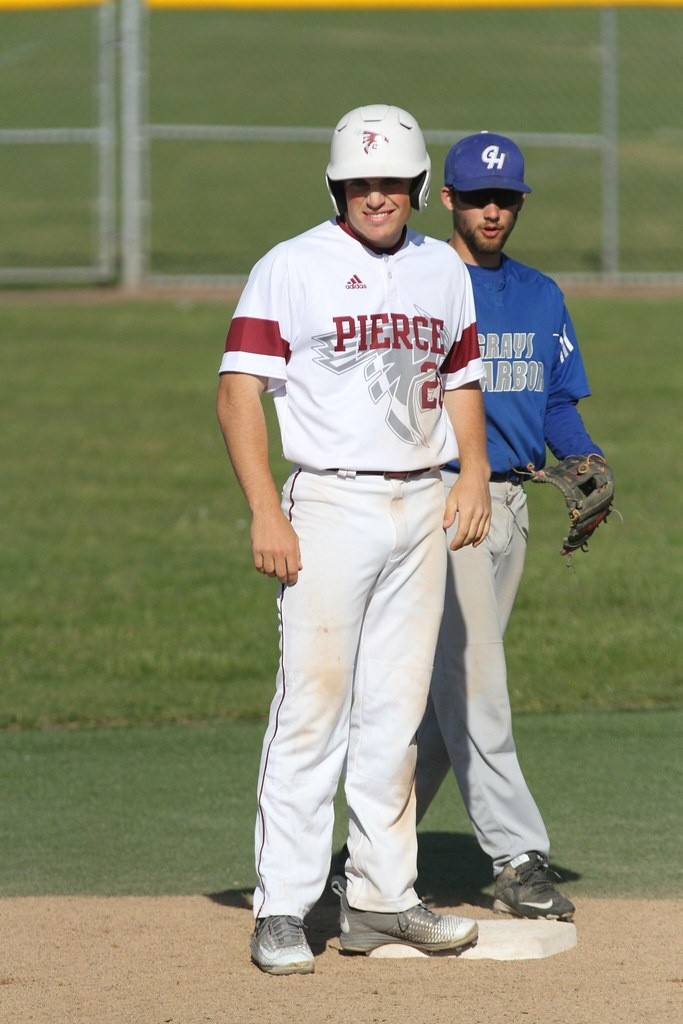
left=450, top=187, right=523, bottom=208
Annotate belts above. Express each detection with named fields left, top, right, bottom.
left=325, top=468, right=431, bottom=480
left=439, top=464, right=521, bottom=486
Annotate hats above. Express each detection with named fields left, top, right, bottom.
left=444, top=131, right=531, bottom=193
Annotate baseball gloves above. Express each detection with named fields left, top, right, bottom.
left=508, top=452, right=624, bottom=574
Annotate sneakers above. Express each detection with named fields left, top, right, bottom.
left=494, top=852, right=576, bottom=921
left=332, top=882, right=480, bottom=954
left=323, top=866, right=349, bottom=903
left=249, top=915, right=315, bottom=976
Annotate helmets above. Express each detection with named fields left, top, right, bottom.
left=325, top=105, right=432, bottom=216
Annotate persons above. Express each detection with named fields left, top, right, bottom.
left=213, top=105, right=492, bottom=973
left=340, top=126, right=615, bottom=923
